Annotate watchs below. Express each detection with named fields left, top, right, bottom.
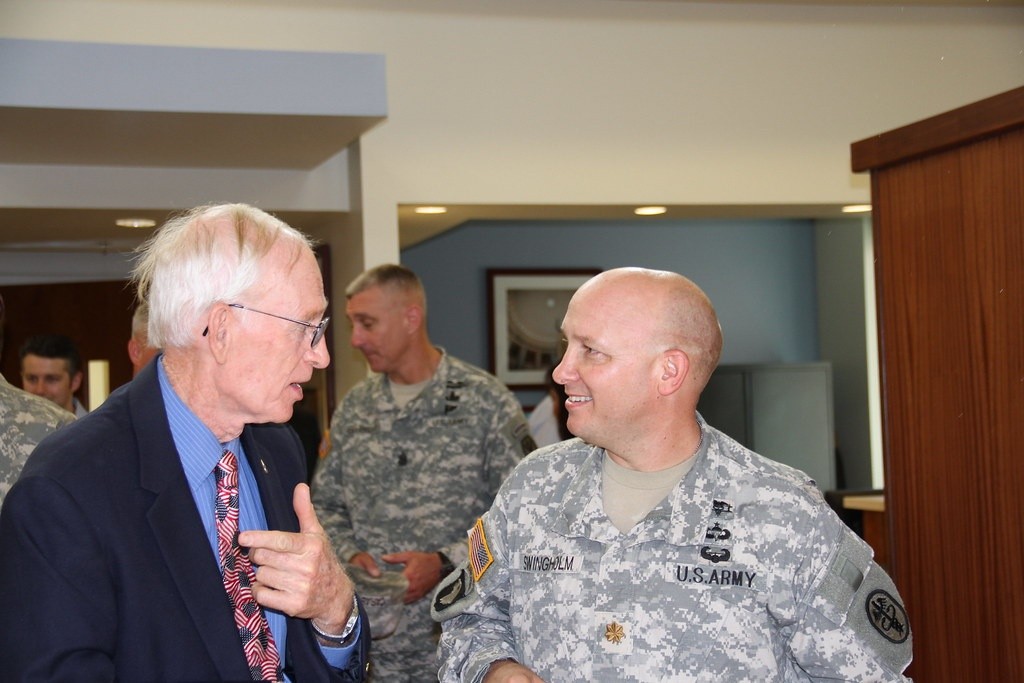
left=311, top=595, right=359, bottom=644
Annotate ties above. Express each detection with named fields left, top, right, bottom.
left=212, top=449, right=284, bottom=683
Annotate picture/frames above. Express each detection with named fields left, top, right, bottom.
left=486, top=266, right=606, bottom=391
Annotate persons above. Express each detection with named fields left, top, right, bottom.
left=311, top=264, right=535, bottom=683
left=527, top=361, right=576, bottom=450
left=18, top=331, right=89, bottom=421
left=0, top=203, right=372, bottom=683
left=431, top=267, right=914, bottom=683
left=128, top=302, right=163, bottom=380
left=0, top=373, right=77, bottom=509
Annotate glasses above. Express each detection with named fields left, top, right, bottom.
left=202, top=304, right=330, bottom=349
